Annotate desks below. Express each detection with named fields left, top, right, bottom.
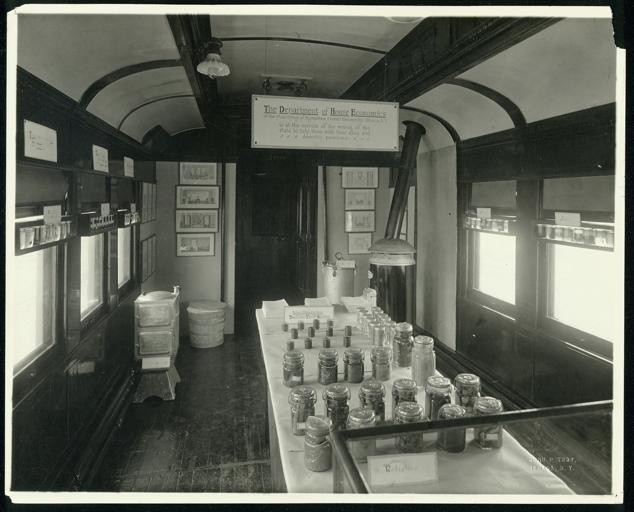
left=253, top=301, right=579, bottom=494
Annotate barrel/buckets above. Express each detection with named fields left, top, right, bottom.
left=323, top=261, right=354, bottom=303
left=186, top=299, right=227, bottom=349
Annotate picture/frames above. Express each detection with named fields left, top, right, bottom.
left=340, top=166, right=380, bottom=254
left=173, top=161, right=222, bottom=258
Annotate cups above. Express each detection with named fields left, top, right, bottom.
left=537, top=223, right=614, bottom=248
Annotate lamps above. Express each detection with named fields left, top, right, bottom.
left=193, top=37, right=232, bottom=78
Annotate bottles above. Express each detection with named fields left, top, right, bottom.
left=124, top=212, right=140, bottom=226
left=19, top=221, right=71, bottom=249
left=470, top=210, right=517, bottom=234
left=281, top=306, right=504, bottom=473
